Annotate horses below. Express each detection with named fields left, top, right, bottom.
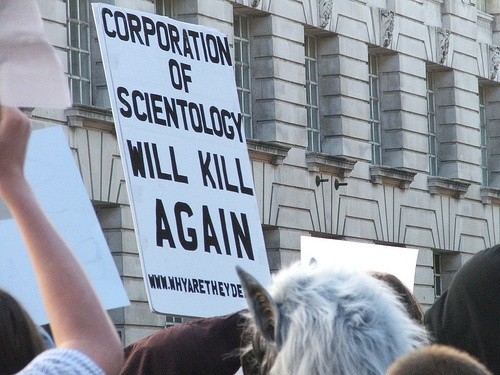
left=237, top=255, right=432, bottom=375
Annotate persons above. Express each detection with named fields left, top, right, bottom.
left=384, top=343, right=493, bottom=375
left=367, top=271, right=424, bottom=320
left=0, top=102, right=124, bottom=375
left=0, top=288, right=47, bottom=375
left=424, top=244, right=500, bottom=375
